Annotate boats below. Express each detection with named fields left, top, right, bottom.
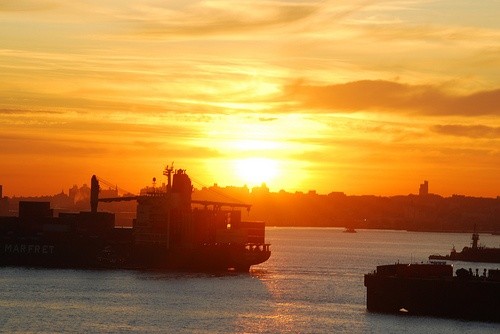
left=0, top=164, right=272, bottom=275
left=428, top=223, right=500, bottom=264
left=362, top=258, right=500, bottom=326
